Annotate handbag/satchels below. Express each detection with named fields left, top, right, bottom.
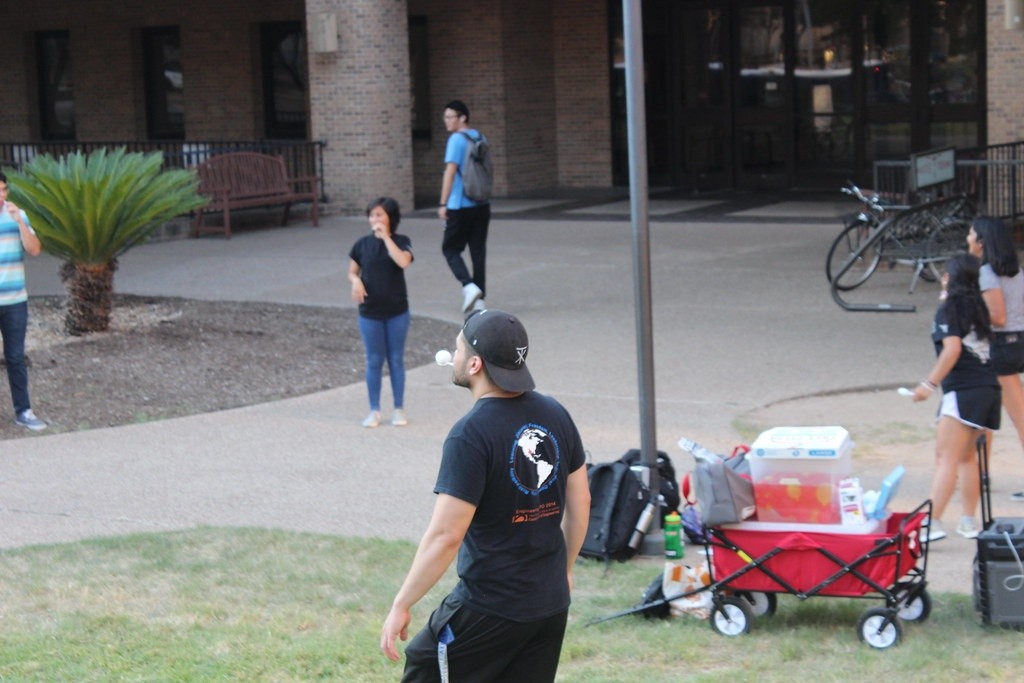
left=644, top=558, right=740, bottom=620
left=689, top=446, right=758, bottom=522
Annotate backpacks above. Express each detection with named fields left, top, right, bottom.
left=572, top=448, right=682, bottom=560
left=455, top=131, right=494, bottom=200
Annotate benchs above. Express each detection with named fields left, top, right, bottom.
left=187, top=150, right=322, bottom=239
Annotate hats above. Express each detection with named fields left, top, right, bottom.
left=463, top=308, right=536, bottom=393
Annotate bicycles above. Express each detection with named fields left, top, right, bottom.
left=824, top=178, right=973, bottom=295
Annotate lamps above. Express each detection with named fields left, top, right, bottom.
left=1005, top=0, right=1024, bottom=29
left=312, top=12, right=340, bottom=53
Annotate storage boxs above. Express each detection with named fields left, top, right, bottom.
left=745, top=424, right=868, bottom=530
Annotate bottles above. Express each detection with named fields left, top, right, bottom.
left=665, top=512, right=684, bottom=560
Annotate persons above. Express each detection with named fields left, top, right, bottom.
left=378, top=307, right=591, bottom=683
left=0, top=167, right=47, bottom=431
left=439, top=100, right=491, bottom=334
left=348, top=197, right=414, bottom=428
left=906, top=215, right=1024, bottom=546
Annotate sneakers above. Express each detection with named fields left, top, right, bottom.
left=15, top=408, right=49, bottom=430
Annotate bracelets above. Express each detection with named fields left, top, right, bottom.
left=921, top=382, right=935, bottom=392
left=924, top=378, right=939, bottom=388
left=440, top=204, right=446, bottom=207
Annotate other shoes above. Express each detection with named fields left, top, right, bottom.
left=462, top=284, right=482, bottom=311
left=391, top=408, right=407, bottom=425
left=920, top=521, right=947, bottom=542
left=464, top=299, right=487, bottom=318
left=957, top=516, right=981, bottom=537
left=362, top=408, right=382, bottom=426
left=1012, top=491, right=1024, bottom=502
